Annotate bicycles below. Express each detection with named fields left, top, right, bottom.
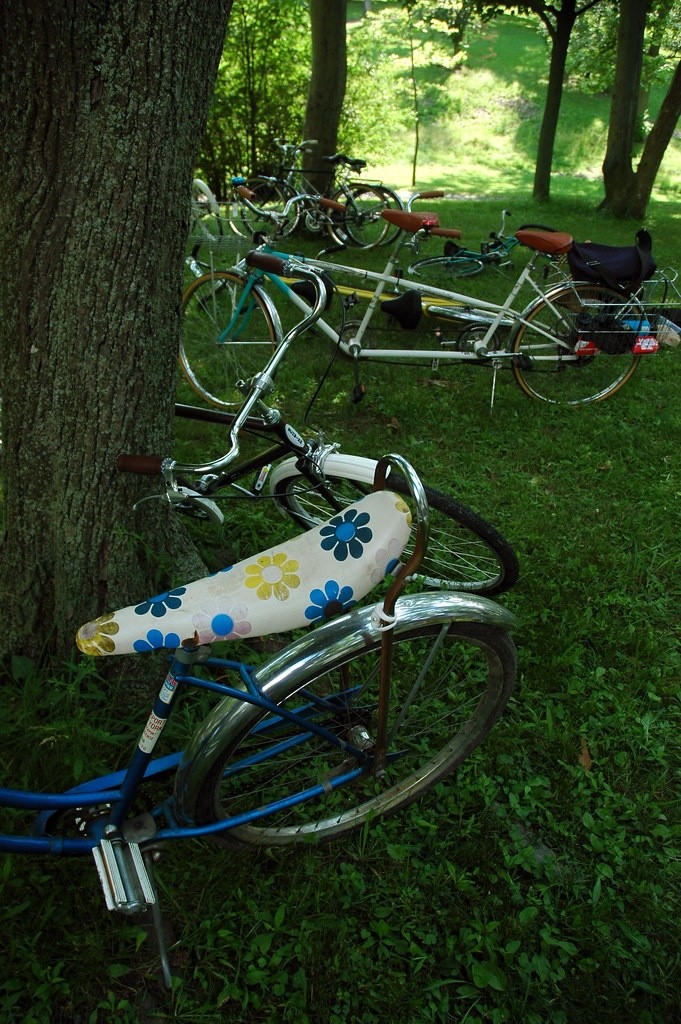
left=174, top=138, right=680, bottom=413
left=112, top=249, right=518, bottom=597
left=0, top=453, right=524, bottom=996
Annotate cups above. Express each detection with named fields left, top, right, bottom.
left=575, top=313, right=592, bottom=340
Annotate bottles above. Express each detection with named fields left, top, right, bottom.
left=621, top=319, right=658, bottom=338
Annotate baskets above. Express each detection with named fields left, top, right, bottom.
left=549, top=262, right=681, bottom=356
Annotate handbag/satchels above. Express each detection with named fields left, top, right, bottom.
left=567, top=228, right=658, bottom=294
